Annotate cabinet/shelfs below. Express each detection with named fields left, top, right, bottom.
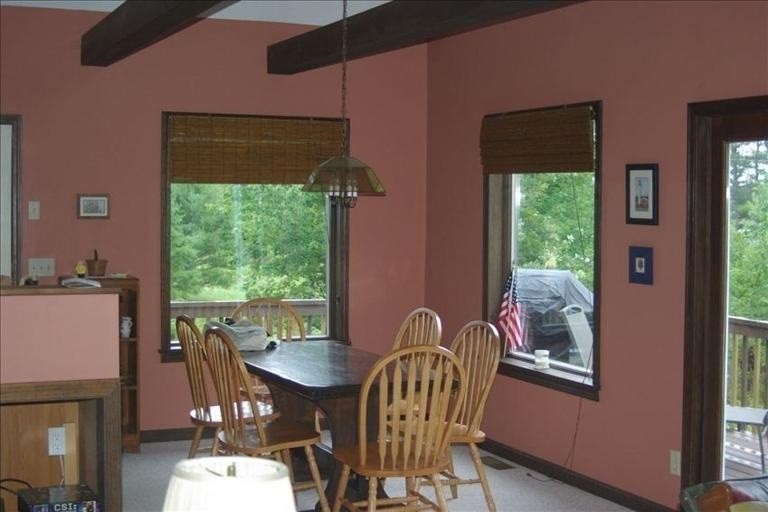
left=65, top=276, right=144, bottom=456
left=0, top=284, right=124, bottom=512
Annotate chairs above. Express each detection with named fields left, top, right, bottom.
left=313, top=334, right=468, bottom=512
left=549, top=301, right=767, bottom=471
left=378, top=307, right=441, bottom=416
left=401, top=312, right=512, bottom=512
left=214, top=293, right=313, bottom=409
left=680, top=467, right=766, bottom=512
left=172, top=309, right=288, bottom=464
left=198, top=325, right=334, bottom=512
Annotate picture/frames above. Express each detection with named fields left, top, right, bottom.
left=623, top=162, right=659, bottom=225
left=75, top=192, right=110, bottom=222
left=0, top=112, right=23, bottom=287
left=627, top=246, right=654, bottom=284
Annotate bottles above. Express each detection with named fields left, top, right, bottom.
left=120, top=316, right=132, bottom=340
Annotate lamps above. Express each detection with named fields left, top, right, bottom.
left=83, top=249, right=107, bottom=276
left=159, top=451, right=302, bottom=512
left=298, top=0, right=388, bottom=208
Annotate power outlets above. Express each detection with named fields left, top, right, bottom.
left=48, top=427, right=64, bottom=457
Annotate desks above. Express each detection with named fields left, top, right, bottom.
left=219, top=329, right=463, bottom=512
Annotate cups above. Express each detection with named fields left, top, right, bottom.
left=534, top=349, right=549, bottom=368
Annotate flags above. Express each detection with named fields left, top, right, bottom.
left=499, top=265, right=524, bottom=357
